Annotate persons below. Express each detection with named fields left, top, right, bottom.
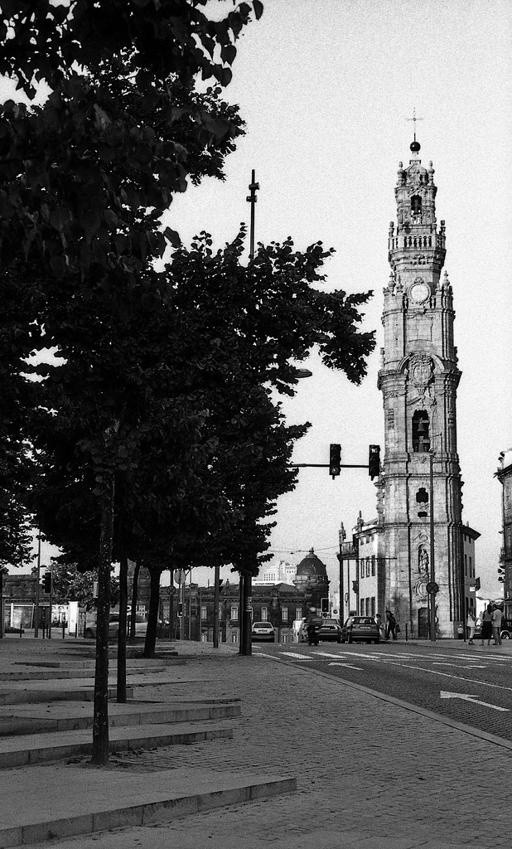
left=491, top=603, right=503, bottom=645
left=467, top=609, right=477, bottom=645
left=376, top=613, right=384, bottom=630
left=304, top=607, right=324, bottom=633
left=385, top=610, right=398, bottom=640
left=479, top=604, right=493, bottom=646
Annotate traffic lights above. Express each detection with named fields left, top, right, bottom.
left=369, top=444, right=379, bottom=475
left=322, top=599, right=329, bottom=612
left=41, top=572, right=51, bottom=593
left=219, top=579, right=224, bottom=591
left=178, top=604, right=185, bottom=616
left=330, top=444, right=342, bottom=475
left=352, top=581, right=358, bottom=592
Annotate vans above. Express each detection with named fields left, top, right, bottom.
left=457, top=618, right=511, bottom=639
left=83, top=613, right=147, bottom=639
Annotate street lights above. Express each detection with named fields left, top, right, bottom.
left=31, top=565, right=47, bottom=576
left=237, top=167, right=260, bottom=658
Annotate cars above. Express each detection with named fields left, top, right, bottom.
left=296, top=618, right=320, bottom=643
left=317, top=618, right=340, bottom=642
left=252, top=621, right=276, bottom=641
left=340, top=616, right=380, bottom=644
